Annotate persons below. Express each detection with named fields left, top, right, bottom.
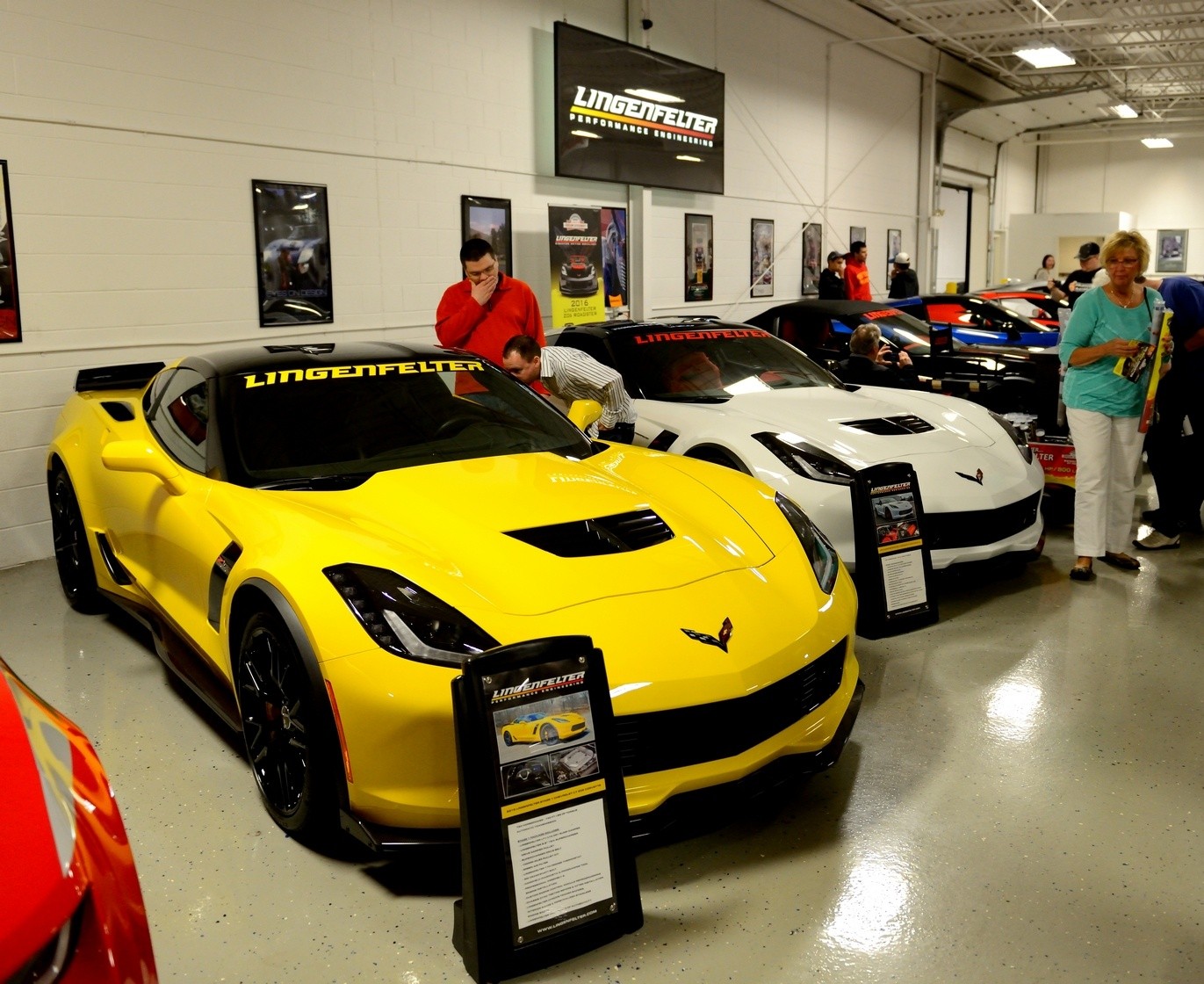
left=1058, top=230, right=1175, bottom=580
left=1034, top=254, right=1055, bottom=282
left=843, top=241, right=872, bottom=302
left=887, top=252, right=919, bottom=299
left=817, top=251, right=850, bottom=300
left=829, top=323, right=919, bottom=390
left=435, top=237, right=551, bottom=415
left=1132, top=275, right=1204, bottom=552
left=502, top=334, right=637, bottom=445
left=1047, top=242, right=1110, bottom=313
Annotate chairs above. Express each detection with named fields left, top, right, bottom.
left=669, top=350, right=723, bottom=393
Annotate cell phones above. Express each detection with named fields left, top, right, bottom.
left=1053, top=280, right=1061, bottom=287
left=885, top=353, right=900, bottom=362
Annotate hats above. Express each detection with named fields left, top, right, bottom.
left=828, top=251, right=845, bottom=262
left=1074, top=242, right=1100, bottom=260
left=889, top=253, right=910, bottom=265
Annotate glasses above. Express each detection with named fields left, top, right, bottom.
left=1107, top=256, right=1140, bottom=267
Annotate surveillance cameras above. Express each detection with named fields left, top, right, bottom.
left=640, top=18, right=653, bottom=31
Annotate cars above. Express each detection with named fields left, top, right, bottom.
left=0, top=659, right=164, bottom=984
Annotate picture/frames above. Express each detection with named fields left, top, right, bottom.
left=593, top=206, right=627, bottom=306
left=685, top=213, right=713, bottom=302
left=1156, top=229, right=1189, bottom=272
left=802, top=223, right=822, bottom=295
left=461, top=195, right=512, bottom=281
left=751, top=218, right=774, bottom=298
left=850, top=226, right=866, bottom=254
left=0, top=159, right=24, bottom=343
left=251, top=179, right=334, bottom=328
left=886, top=228, right=901, bottom=289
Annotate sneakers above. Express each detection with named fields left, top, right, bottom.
left=1142, top=509, right=1161, bottom=523
left=1132, top=530, right=1182, bottom=551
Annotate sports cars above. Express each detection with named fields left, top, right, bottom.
left=544, top=315, right=1047, bottom=578
left=502, top=711, right=587, bottom=746
left=44, top=339, right=863, bottom=864
left=558, top=255, right=598, bottom=296
left=742, top=280, right=1075, bottom=529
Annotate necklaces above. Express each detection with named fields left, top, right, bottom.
left=1108, top=282, right=1134, bottom=309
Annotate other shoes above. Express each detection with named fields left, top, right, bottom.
left=1097, top=550, right=1141, bottom=570
left=1068, top=558, right=1094, bottom=581
